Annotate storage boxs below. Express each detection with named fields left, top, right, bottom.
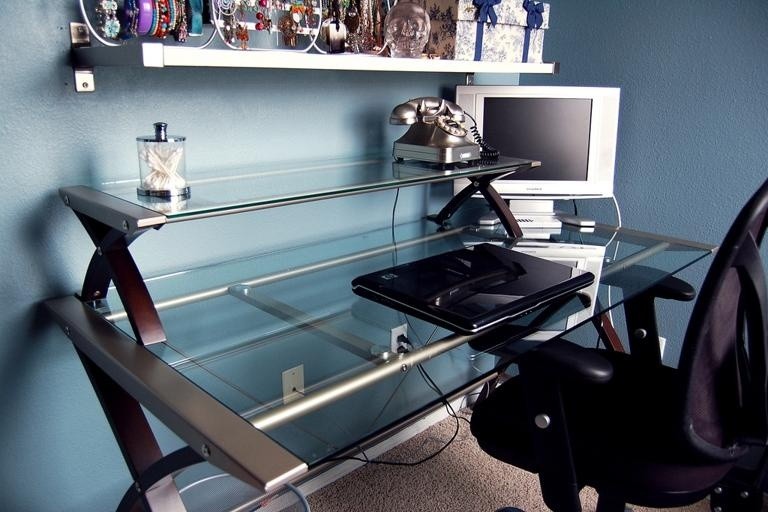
left=412, top=0, right=552, bottom=62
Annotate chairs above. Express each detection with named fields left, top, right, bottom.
left=468, top=181, right=767, bottom=511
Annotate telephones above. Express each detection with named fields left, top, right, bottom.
left=390, top=96, right=484, bottom=171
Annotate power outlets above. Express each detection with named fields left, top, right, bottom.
left=389, top=323, right=408, bottom=363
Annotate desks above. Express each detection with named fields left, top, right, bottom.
left=43, top=140, right=721, bottom=512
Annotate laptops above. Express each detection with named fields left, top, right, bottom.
left=351, top=242, right=594, bottom=336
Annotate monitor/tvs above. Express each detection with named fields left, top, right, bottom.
left=450, top=225, right=606, bottom=342
left=453, top=85, right=621, bottom=227
